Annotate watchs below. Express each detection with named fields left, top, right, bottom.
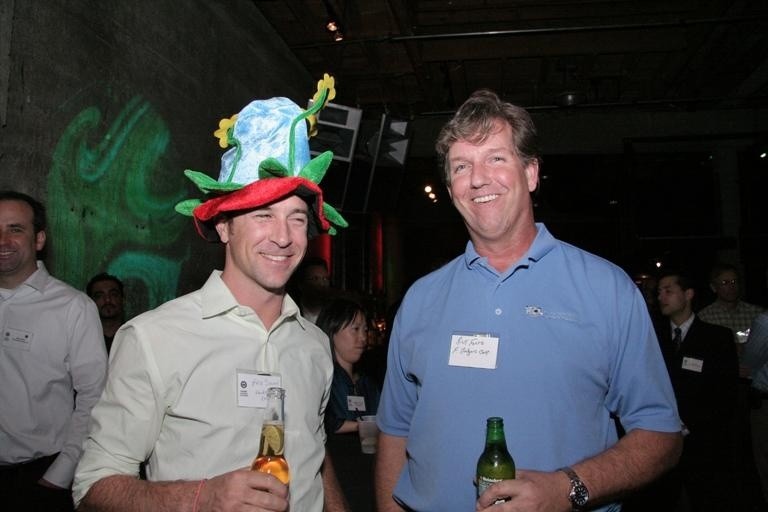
left=555, top=466, right=590, bottom=511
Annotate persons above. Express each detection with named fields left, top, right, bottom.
left=86, top=271, right=127, bottom=356
left=0, top=188, right=108, bottom=512
left=735, top=309, right=768, bottom=511
left=622, top=266, right=746, bottom=511
left=695, top=265, right=762, bottom=379
left=373, top=89, right=683, bottom=511
left=70, top=93, right=348, bottom=512
left=290, top=249, right=423, bottom=511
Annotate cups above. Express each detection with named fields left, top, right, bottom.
left=355, top=416, right=380, bottom=456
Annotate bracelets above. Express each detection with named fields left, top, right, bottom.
left=192, top=481, right=205, bottom=512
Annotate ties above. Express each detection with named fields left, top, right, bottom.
left=671, top=326, right=682, bottom=354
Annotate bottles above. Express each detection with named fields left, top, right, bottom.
left=251, top=386, right=290, bottom=507
left=477, top=416, right=516, bottom=506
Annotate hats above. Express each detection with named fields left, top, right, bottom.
left=175, top=73, right=351, bottom=243
